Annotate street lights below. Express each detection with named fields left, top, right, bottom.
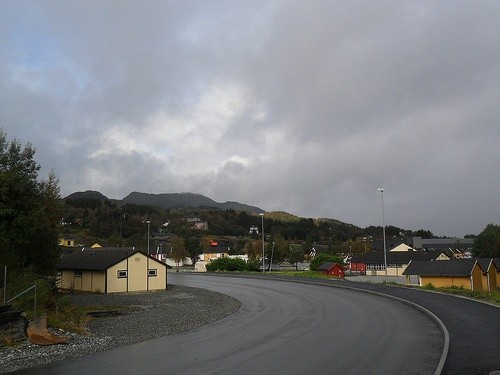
left=260, top=213, right=265, bottom=274
left=376, top=184, right=388, bottom=268
left=146, top=221, right=150, bottom=255
